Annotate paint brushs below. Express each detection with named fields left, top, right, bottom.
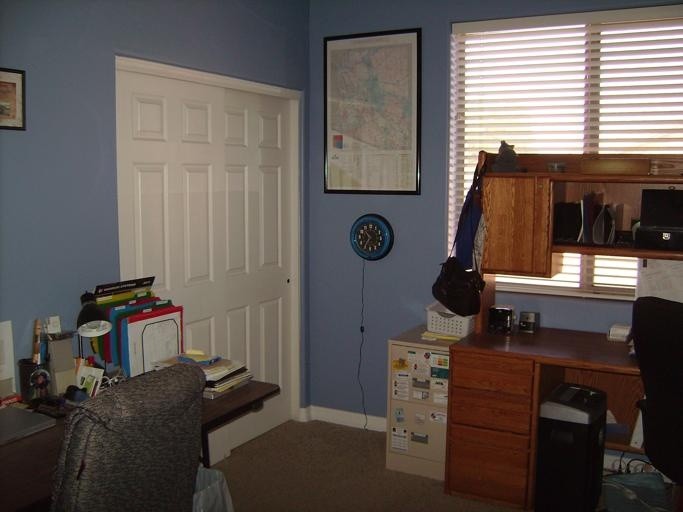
left=32, top=320, right=48, bottom=365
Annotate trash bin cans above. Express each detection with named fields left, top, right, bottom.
left=535, top=381, right=608, bottom=512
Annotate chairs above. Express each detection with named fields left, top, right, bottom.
left=47, top=358, right=207, bottom=512
left=631, top=297, right=683, bottom=483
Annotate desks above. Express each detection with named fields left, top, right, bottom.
left=444, top=328, right=642, bottom=512
left=0, top=379, right=282, bottom=512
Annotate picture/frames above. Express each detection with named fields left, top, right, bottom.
left=0, top=67, right=27, bottom=131
left=324, top=27, right=421, bottom=195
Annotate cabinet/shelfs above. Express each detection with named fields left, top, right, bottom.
left=385, top=325, right=461, bottom=480
left=475, top=150, right=683, bottom=278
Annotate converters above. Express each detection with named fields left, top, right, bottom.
left=622, top=487, right=637, bottom=501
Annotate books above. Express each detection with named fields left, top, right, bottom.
left=154, top=351, right=254, bottom=400
left=540, top=383, right=607, bottom=425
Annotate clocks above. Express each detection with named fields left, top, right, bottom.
left=350, top=214, right=394, bottom=261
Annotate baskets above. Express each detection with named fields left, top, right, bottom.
left=424, top=300, right=475, bottom=337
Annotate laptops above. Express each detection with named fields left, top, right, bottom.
left=0, top=406, right=57, bottom=446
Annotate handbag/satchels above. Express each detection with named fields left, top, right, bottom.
left=432, top=232, right=485, bottom=317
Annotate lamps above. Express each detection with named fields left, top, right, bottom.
left=78, top=291, right=112, bottom=357
